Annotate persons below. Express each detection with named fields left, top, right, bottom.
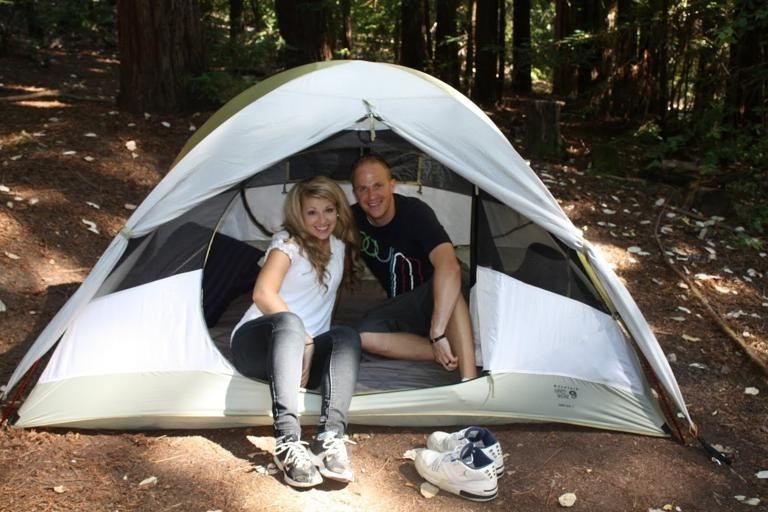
left=229, top=174, right=363, bottom=489
left=348, top=152, right=478, bottom=383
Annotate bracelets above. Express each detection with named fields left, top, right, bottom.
left=430, top=331, right=447, bottom=346
left=305, top=342, right=316, bottom=347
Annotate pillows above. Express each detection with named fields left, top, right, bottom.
left=118, top=221, right=266, bottom=327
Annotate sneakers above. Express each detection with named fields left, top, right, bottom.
left=414, top=444, right=498, bottom=502
left=307, top=431, right=357, bottom=483
left=272, top=434, right=322, bottom=488
left=426, top=426, right=505, bottom=479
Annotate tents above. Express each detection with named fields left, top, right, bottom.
left=0, top=57, right=697, bottom=446
